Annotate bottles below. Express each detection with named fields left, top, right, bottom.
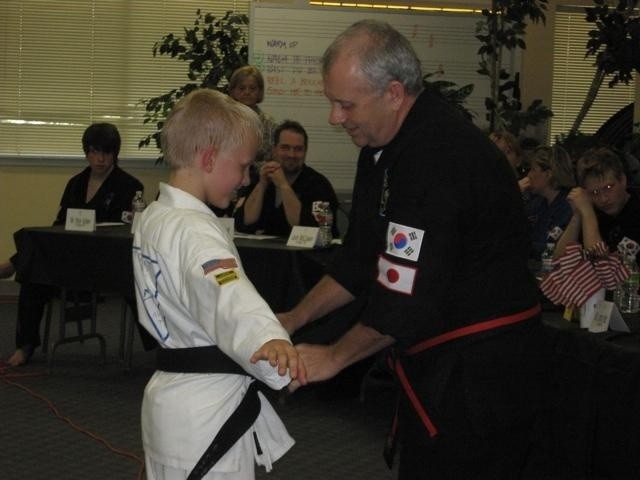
left=541, top=243, right=561, bottom=273
left=318, top=202, right=333, bottom=248
left=620, top=253, right=640, bottom=314
left=131, top=190, right=144, bottom=223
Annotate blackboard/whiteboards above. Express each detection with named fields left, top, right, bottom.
left=248, top=4, right=503, bottom=197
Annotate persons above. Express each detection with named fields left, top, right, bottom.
left=486, top=129, right=640, bottom=269
left=227, top=66, right=277, bottom=204
left=132, top=89, right=309, bottom=480
left=233, top=119, right=340, bottom=239
left=0, top=122, right=144, bottom=367
left=275, top=20, right=544, bottom=478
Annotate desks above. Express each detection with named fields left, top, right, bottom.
left=12, top=224, right=343, bottom=368
